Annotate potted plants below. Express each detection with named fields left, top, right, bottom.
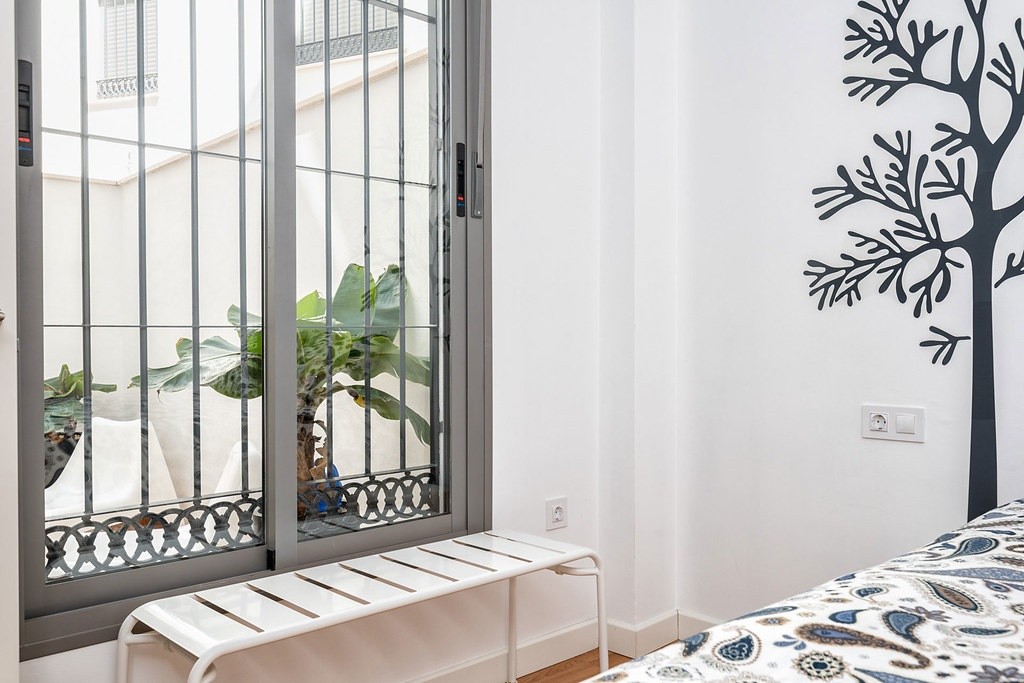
left=125, top=262, right=434, bottom=517
left=44, top=365, right=117, bottom=490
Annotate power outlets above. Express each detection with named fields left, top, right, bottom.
left=545, top=496, right=567, bottom=530
left=860, top=405, right=926, bottom=443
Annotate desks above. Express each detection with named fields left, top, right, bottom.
left=117, top=530, right=608, bottom=683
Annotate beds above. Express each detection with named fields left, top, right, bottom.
left=572, top=496, right=1024, bottom=683
